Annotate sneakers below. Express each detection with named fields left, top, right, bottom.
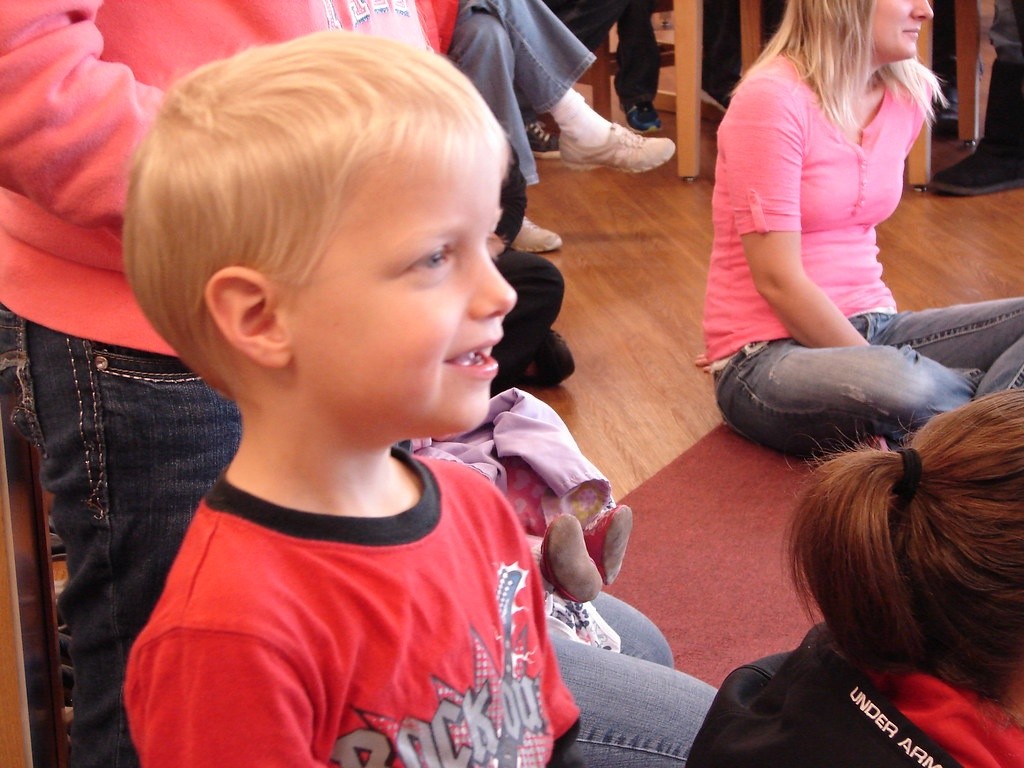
left=559, top=122, right=676, bottom=175
left=701, top=69, right=732, bottom=111
left=511, top=216, right=563, bottom=253
left=613, top=75, right=664, bottom=131
left=525, top=121, right=561, bottom=158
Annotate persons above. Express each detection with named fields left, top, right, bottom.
left=123, top=32, right=581, bottom=768
left=1, top=1, right=1024, bottom=768
left=694, top=0, right=1024, bottom=460
left=683, top=388, right=1024, bottom=768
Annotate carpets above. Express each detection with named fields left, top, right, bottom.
left=602, top=421, right=826, bottom=688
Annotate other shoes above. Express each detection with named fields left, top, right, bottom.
left=930, top=151, right=1024, bottom=195
left=538, top=504, right=634, bottom=605
left=536, top=328, right=575, bottom=386
